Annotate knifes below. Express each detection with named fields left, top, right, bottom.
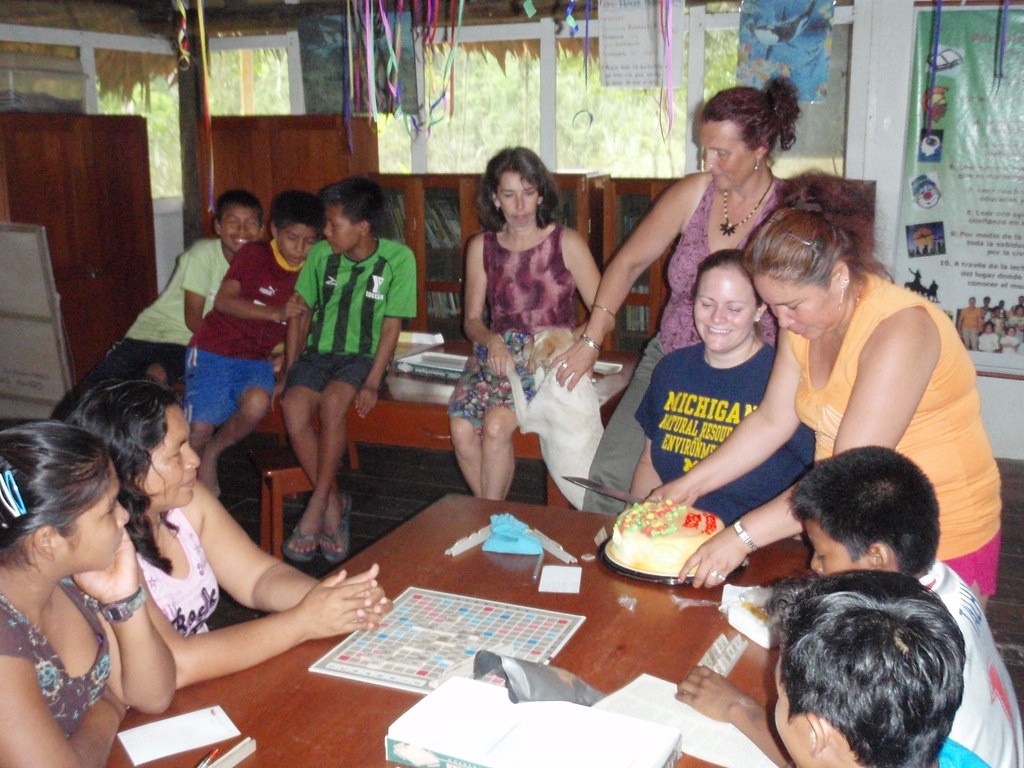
left=562, top=476, right=642, bottom=504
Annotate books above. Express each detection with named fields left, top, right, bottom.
left=384, top=195, right=650, bottom=332
left=384, top=676, right=682, bottom=768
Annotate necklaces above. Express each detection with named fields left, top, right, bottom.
left=856, top=280, right=863, bottom=306
left=720, top=175, right=774, bottom=236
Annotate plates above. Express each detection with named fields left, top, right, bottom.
left=596, top=536, right=750, bottom=586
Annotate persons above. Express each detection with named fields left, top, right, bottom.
left=0, top=420, right=177, bottom=768
left=623, top=249, right=816, bottom=541
left=549, top=76, right=800, bottom=515
left=645, top=168, right=1002, bottom=616
left=674, top=445, right=1024, bottom=768
left=79, top=188, right=265, bottom=391
left=183, top=190, right=324, bottom=499
left=765, top=571, right=966, bottom=768
left=446, top=147, right=602, bottom=500
left=270, top=176, right=417, bottom=561
left=958, top=296, right=1024, bottom=355
left=62, top=376, right=395, bottom=690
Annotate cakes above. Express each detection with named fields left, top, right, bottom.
left=610, top=499, right=725, bottom=577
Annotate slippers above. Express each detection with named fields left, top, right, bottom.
left=282, top=516, right=324, bottom=563
left=318, top=492, right=354, bottom=564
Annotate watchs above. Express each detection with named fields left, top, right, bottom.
left=100, top=586, right=146, bottom=624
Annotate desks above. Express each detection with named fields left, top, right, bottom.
left=253, top=341, right=651, bottom=509
left=104, top=490, right=820, bottom=768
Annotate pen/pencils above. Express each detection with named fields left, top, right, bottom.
left=531, top=552, right=546, bottom=580
left=197, top=747, right=220, bottom=768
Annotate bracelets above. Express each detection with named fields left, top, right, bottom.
left=592, top=304, right=616, bottom=317
left=581, top=334, right=601, bottom=352
left=734, top=519, right=760, bottom=552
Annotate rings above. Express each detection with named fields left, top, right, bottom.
left=719, top=575, right=726, bottom=581
left=711, top=569, right=718, bottom=577
left=562, top=362, right=568, bottom=369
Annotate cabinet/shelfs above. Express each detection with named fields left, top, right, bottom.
left=366, top=168, right=590, bottom=350
left=593, top=176, right=879, bottom=357
left=0, top=111, right=160, bottom=383
left=196, top=112, right=375, bottom=234
